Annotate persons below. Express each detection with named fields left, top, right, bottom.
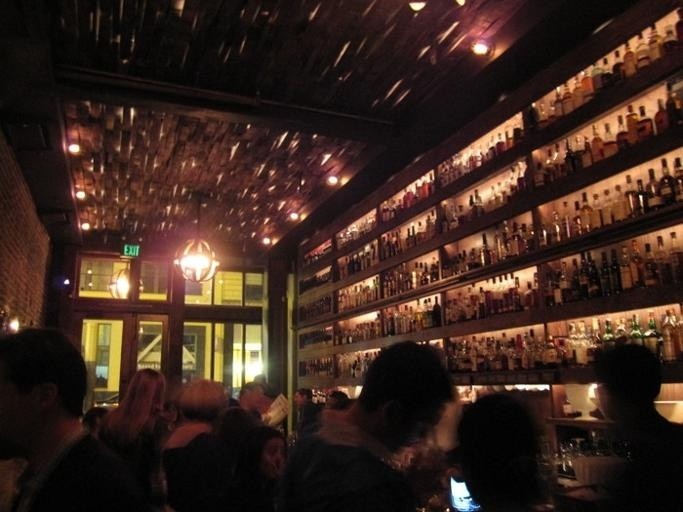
left=0, top=327, right=682, bottom=512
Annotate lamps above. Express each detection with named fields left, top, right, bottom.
left=106, top=255, right=144, bottom=299
left=174, top=188, right=221, bottom=282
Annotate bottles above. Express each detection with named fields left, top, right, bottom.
left=296, top=8, right=680, bottom=482
